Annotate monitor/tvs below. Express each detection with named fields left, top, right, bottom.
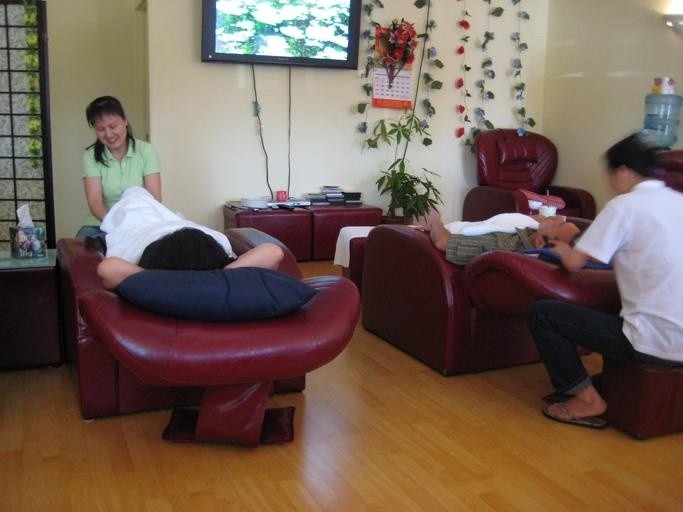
left=201, top=0, right=363, bottom=70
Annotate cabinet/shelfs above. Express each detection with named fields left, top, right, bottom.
left=0, top=249, right=63, bottom=371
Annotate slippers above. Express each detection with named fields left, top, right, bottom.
left=544, top=403, right=606, bottom=429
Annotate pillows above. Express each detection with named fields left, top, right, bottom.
left=115, top=266, right=320, bottom=323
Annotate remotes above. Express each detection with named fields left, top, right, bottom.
left=278, top=204, right=294, bottom=212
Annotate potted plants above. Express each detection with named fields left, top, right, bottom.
left=366, top=111, right=449, bottom=226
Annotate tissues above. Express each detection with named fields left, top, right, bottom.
left=7, top=204, right=46, bottom=259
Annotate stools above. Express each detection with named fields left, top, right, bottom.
left=302, top=197, right=383, bottom=261
left=222, top=200, right=313, bottom=264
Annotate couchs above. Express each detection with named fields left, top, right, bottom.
left=574, top=342, right=683, bottom=441
left=340, top=212, right=623, bottom=378
left=54, top=225, right=362, bottom=448
left=462, top=127, right=597, bottom=224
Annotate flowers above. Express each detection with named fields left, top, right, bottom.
left=427, top=48, right=437, bottom=60
left=515, top=127, right=529, bottom=139
left=372, top=18, right=417, bottom=90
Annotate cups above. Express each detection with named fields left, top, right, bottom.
left=537, top=205, right=558, bottom=218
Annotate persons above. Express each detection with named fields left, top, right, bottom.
left=97, top=229, right=283, bottom=291
left=80, top=97, right=161, bottom=221
left=529, top=130, right=683, bottom=429
left=334, top=206, right=580, bottom=266
